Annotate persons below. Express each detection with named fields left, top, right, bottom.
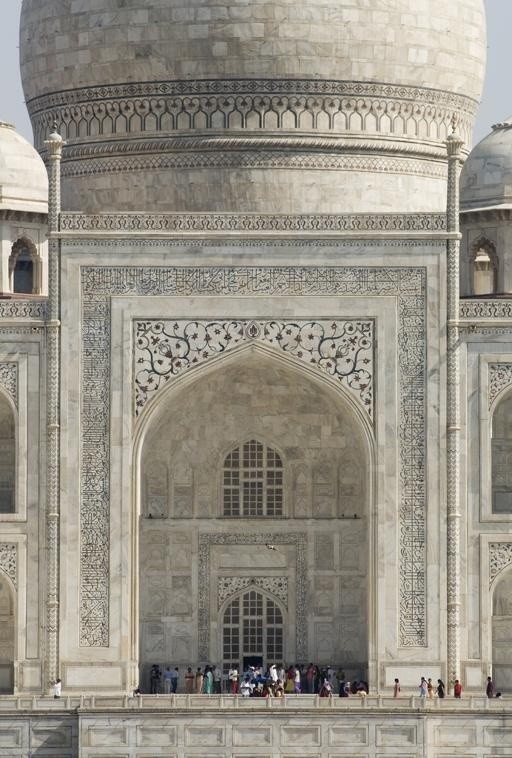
left=419, top=677, right=427, bottom=697
left=393, top=678, right=400, bottom=697
left=434, top=679, right=445, bottom=698
left=427, top=678, right=434, bottom=697
left=53, top=678, right=61, bottom=698
left=486, top=676, right=494, bottom=698
left=149, top=662, right=368, bottom=698
left=454, top=679, right=463, bottom=698
left=494, top=691, right=503, bottom=699
